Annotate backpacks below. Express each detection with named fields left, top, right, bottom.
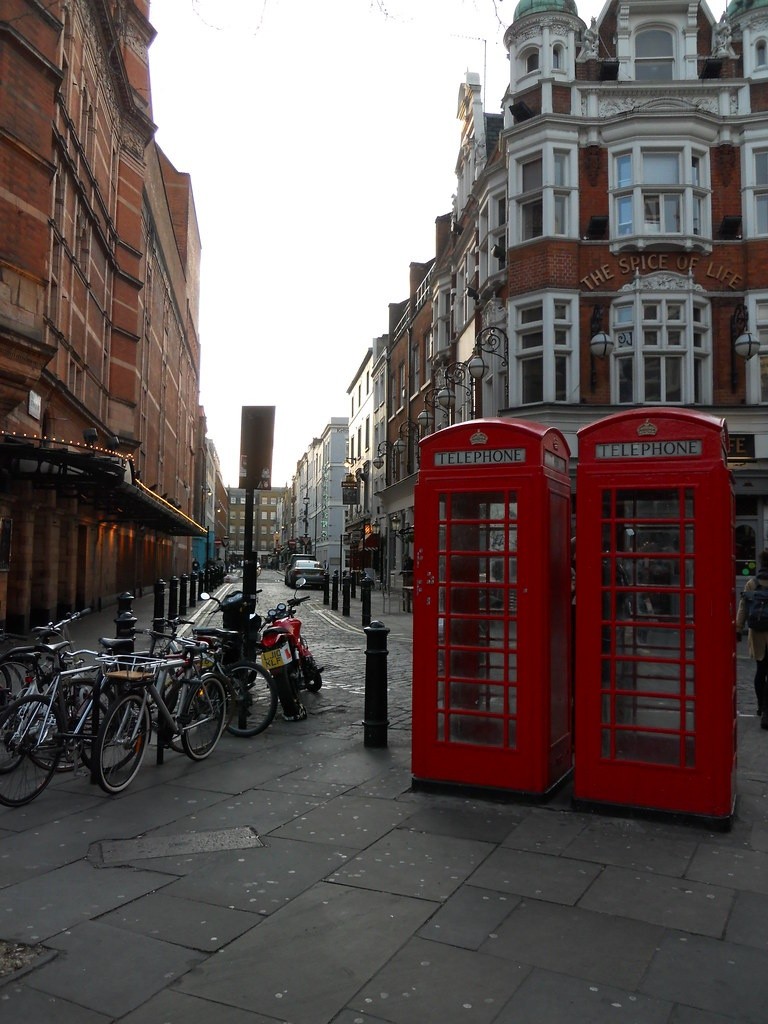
left=740, top=576, right=768, bottom=633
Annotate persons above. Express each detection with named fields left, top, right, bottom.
left=735, top=551, right=768, bottom=728
left=400, top=554, right=413, bottom=600
left=192, top=557, right=230, bottom=579
left=566, top=532, right=672, bottom=725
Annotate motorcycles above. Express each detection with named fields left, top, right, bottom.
left=246, top=575, right=326, bottom=719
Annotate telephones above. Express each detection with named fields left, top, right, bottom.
left=480, top=555, right=520, bottom=614
left=603, top=551, right=630, bottom=618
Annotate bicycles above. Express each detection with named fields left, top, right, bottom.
left=0, top=604, right=278, bottom=807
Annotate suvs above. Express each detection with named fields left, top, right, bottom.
left=284, top=554, right=318, bottom=585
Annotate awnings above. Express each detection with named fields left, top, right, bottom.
left=358, top=534, right=380, bottom=550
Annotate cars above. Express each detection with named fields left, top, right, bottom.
left=287, top=559, right=325, bottom=590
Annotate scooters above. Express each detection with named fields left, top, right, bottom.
left=199, top=588, right=262, bottom=731
